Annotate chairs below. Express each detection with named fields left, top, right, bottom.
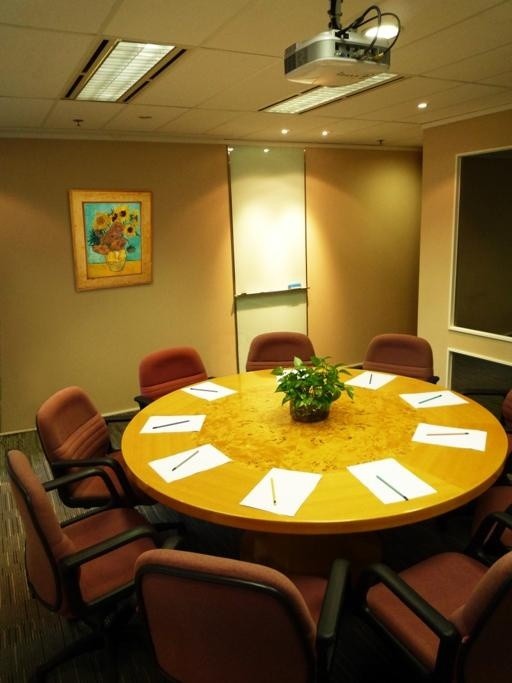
left=34, top=385, right=158, bottom=510
left=467, top=505, right=512, bottom=563
left=7, top=449, right=161, bottom=613
left=132, top=347, right=210, bottom=410
left=246, top=334, right=316, bottom=370
left=489, top=392, right=512, bottom=492
left=360, top=532, right=512, bottom=683
left=342, top=332, right=441, bottom=384
left=133, top=542, right=351, bottom=682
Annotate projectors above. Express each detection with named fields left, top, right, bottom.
left=283, top=29, right=391, bottom=87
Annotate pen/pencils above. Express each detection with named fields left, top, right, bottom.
left=270, top=477, right=277, bottom=504
left=427, top=433, right=469, bottom=435
left=376, top=476, right=409, bottom=501
left=190, top=388, right=219, bottom=392
left=369, top=374, right=373, bottom=384
left=153, top=420, right=191, bottom=429
left=419, top=395, right=442, bottom=404
left=172, top=450, right=199, bottom=471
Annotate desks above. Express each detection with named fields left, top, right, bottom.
left=129, top=364, right=509, bottom=569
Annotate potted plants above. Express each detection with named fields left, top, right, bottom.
left=274, top=355, right=348, bottom=421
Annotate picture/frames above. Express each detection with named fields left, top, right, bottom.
left=448, top=146, right=512, bottom=342
left=69, top=189, right=152, bottom=292
